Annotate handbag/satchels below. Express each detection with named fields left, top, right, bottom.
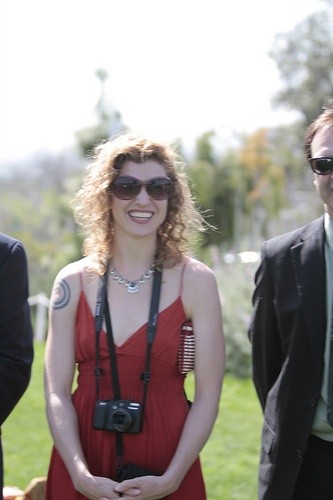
left=117, top=465, right=163, bottom=497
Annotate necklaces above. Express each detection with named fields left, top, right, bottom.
left=101, top=243, right=167, bottom=293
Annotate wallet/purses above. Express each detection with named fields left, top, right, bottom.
left=177, top=321, right=194, bottom=374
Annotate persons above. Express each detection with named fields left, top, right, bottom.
left=0, top=230, right=34, bottom=498
left=246, top=105, right=333, bottom=500
left=39, top=132, right=227, bottom=500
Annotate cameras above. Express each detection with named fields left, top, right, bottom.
left=92, top=399, right=142, bottom=434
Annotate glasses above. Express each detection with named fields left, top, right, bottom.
left=107, top=176, right=174, bottom=200
left=307, top=157, right=333, bottom=175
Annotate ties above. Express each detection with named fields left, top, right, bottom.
left=326, top=317, right=332, bottom=428
